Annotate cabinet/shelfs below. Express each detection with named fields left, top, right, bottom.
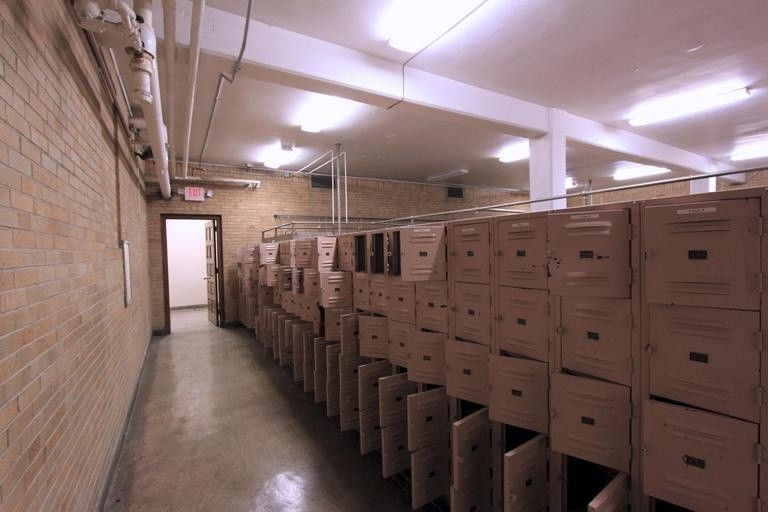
left=237, top=186, right=768, bottom=512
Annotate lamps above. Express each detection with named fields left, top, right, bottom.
left=628, top=86, right=753, bottom=130
